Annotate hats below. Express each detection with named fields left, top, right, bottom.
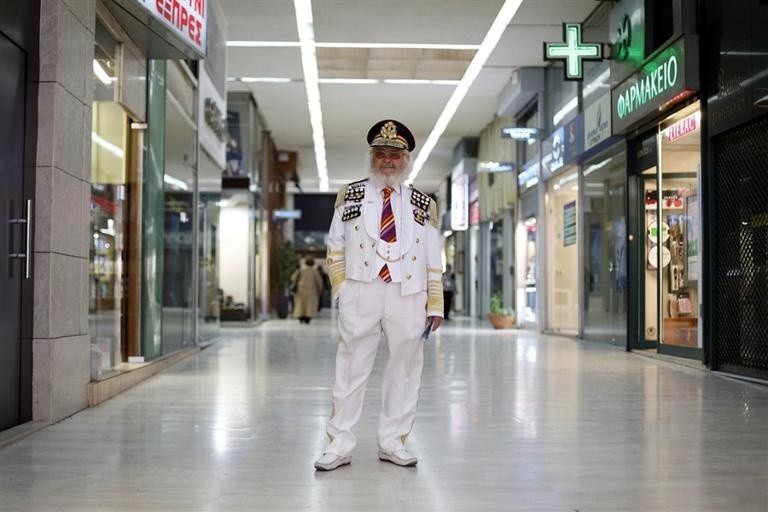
left=367, top=120, right=415, bottom=152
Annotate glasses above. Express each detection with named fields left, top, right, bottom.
left=375, top=152, right=401, bottom=160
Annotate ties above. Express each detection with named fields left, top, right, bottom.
left=380, top=185, right=397, bottom=284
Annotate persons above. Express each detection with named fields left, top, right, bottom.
left=288, top=254, right=331, bottom=324
left=314, top=119, right=445, bottom=472
left=442, top=263, right=459, bottom=321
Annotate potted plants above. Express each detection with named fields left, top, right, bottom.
left=489, top=293, right=514, bottom=330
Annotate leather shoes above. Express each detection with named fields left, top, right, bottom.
left=378, top=449, right=418, bottom=466
left=314, top=453, right=352, bottom=471
